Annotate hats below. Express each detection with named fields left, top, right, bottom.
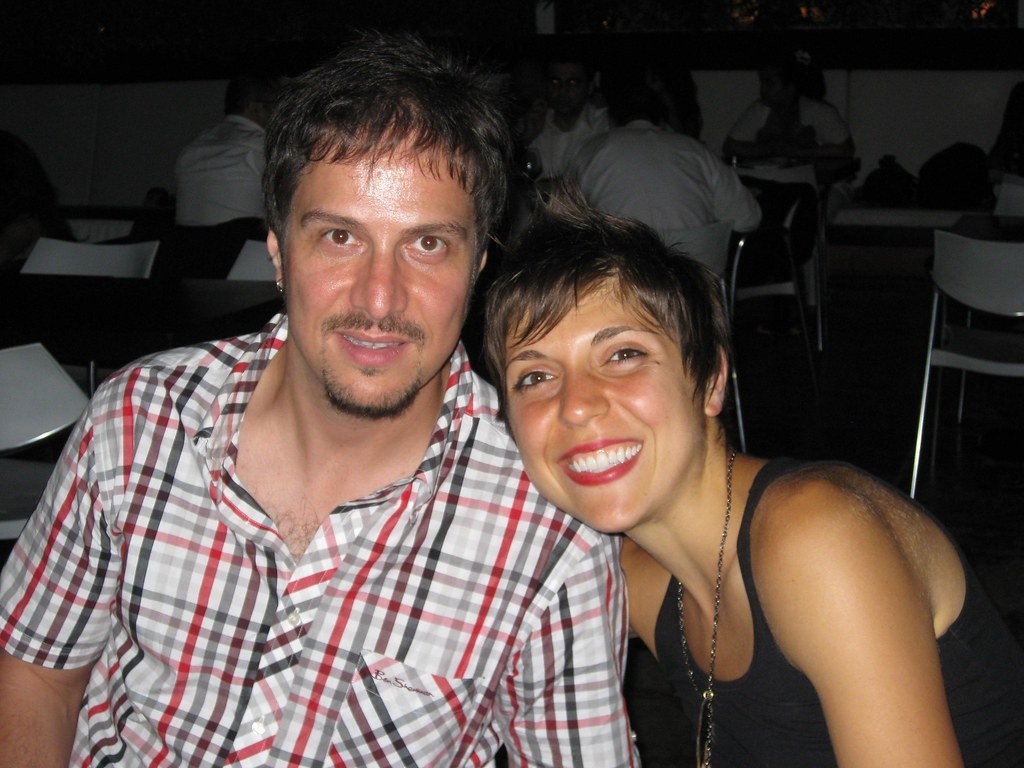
left=748, top=42, right=821, bottom=73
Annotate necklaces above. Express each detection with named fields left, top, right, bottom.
left=677, top=448, right=739, bottom=768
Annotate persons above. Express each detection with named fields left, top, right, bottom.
left=0, top=37, right=644, bottom=768
left=503, top=51, right=857, bottom=336
left=174, top=67, right=286, bottom=226
left=0, top=127, right=79, bottom=266
left=966, top=80, right=1024, bottom=469
left=477, top=218, right=1024, bottom=768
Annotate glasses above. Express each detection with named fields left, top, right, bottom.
left=545, top=77, right=582, bottom=91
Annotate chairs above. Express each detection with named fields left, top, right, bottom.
left=1, top=138, right=1024, bottom=575
